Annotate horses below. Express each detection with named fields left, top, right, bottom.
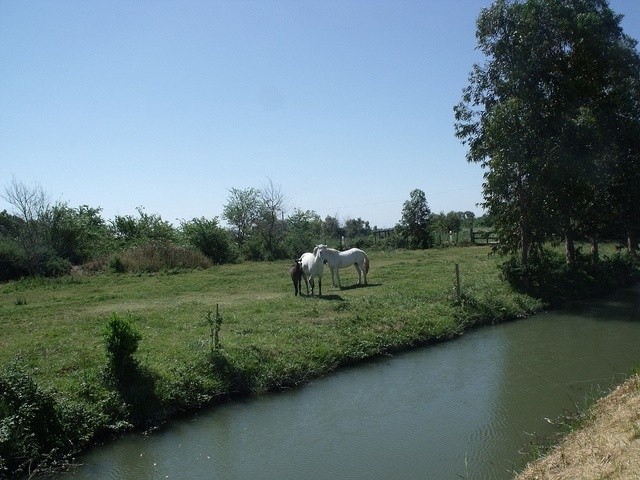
left=290, top=258, right=303, bottom=296
left=324, top=247, right=369, bottom=288
left=298, top=244, right=328, bottom=297
